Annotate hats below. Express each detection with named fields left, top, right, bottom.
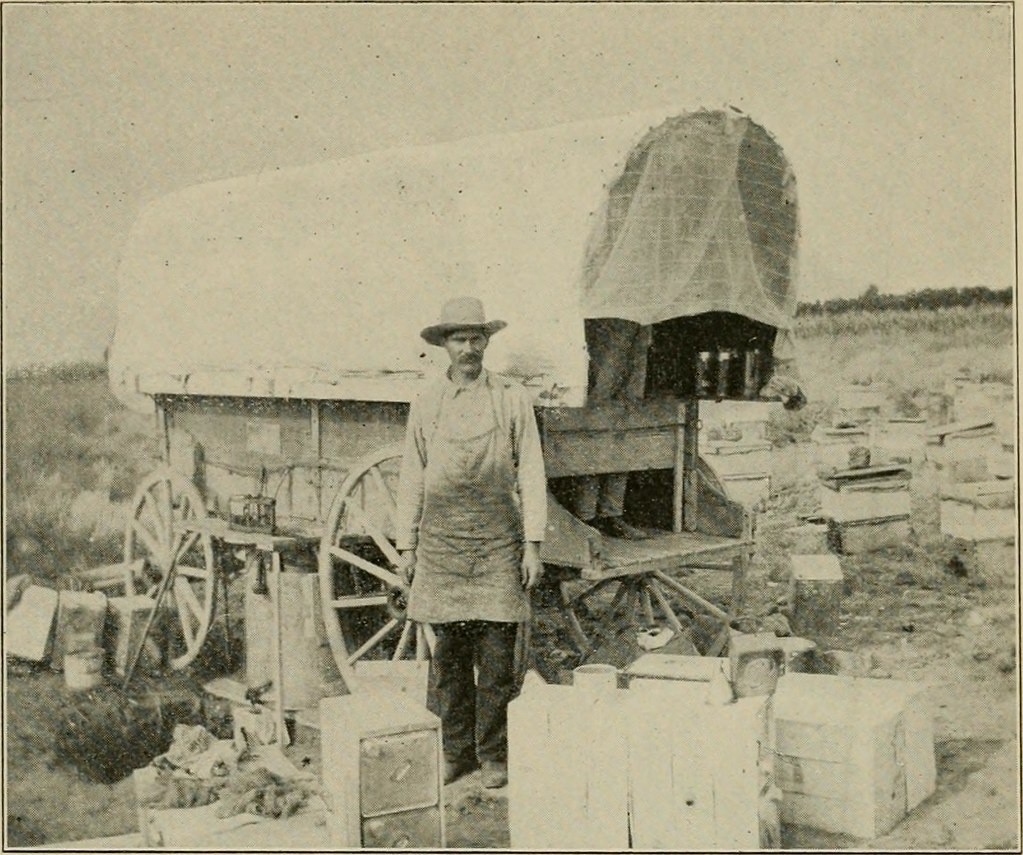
left=419, top=296, right=508, bottom=347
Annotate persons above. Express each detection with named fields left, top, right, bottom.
left=396, top=297, right=547, bottom=790
left=573, top=321, right=650, bottom=540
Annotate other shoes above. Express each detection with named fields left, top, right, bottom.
left=480, top=760, right=508, bottom=787
left=605, top=516, right=645, bottom=538
left=592, top=519, right=609, bottom=536
left=444, top=761, right=472, bottom=785
